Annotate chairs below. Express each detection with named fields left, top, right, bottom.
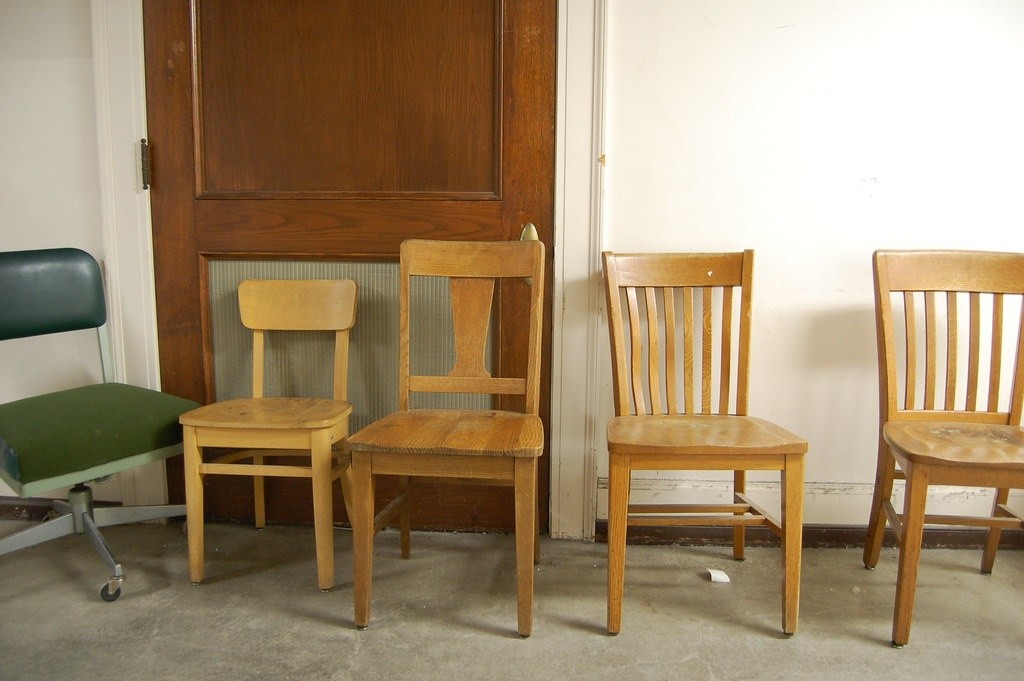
left=346, top=240, right=546, bottom=638
left=180, top=280, right=360, bottom=591
left=602, top=250, right=809, bottom=639
left=863, top=248, right=1024, bottom=648
left=0, top=249, right=203, bottom=602
